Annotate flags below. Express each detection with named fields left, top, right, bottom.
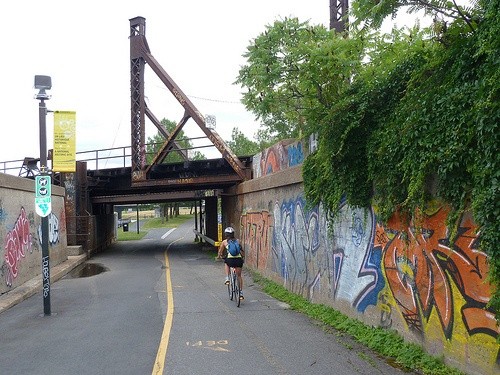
left=53, top=110, right=77, bottom=172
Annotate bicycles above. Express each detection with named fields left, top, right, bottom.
left=219, top=254, right=243, bottom=308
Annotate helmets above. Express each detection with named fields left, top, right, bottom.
left=225, top=226, right=235, bottom=234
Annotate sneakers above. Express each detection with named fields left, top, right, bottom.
left=240, top=294, right=244, bottom=300
left=224, top=277, right=230, bottom=285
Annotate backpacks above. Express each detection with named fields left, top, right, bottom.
left=226, top=238, right=241, bottom=256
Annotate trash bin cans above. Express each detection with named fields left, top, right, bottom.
left=123, top=222, right=128, bottom=232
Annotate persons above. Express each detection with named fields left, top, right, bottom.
left=216, top=226, right=245, bottom=299
